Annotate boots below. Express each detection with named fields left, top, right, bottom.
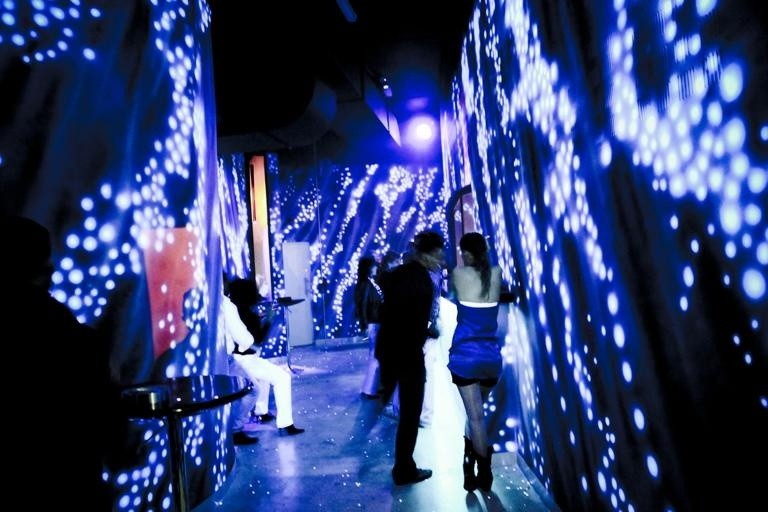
left=461, top=435, right=478, bottom=493
left=476, top=445, right=493, bottom=492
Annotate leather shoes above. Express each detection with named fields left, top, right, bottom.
left=360, top=391, right=378, bottom=400
left=230, top=430, right=260, bottom=445
left=392, top=466, right=433, bottom=484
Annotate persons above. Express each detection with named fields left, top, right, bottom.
left=223, top=282, right=260, bottom=449
left=0, top=214, right=139, bottom=510
left=372, top=228, right=446, bottom=486
left=376, top=249, right=402, bottom=294
left=227, top=278, right=307, bottom=438
left=449, top=231, right=505, bottom=495
left=352, top=253, right=389, bottom=401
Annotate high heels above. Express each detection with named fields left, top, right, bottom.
left=279, top=424, right=306, bottom=438
left=255, top=411, right=276, bottom=425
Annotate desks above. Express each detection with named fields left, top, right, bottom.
left=119, top=374, right=252, bottom=511
